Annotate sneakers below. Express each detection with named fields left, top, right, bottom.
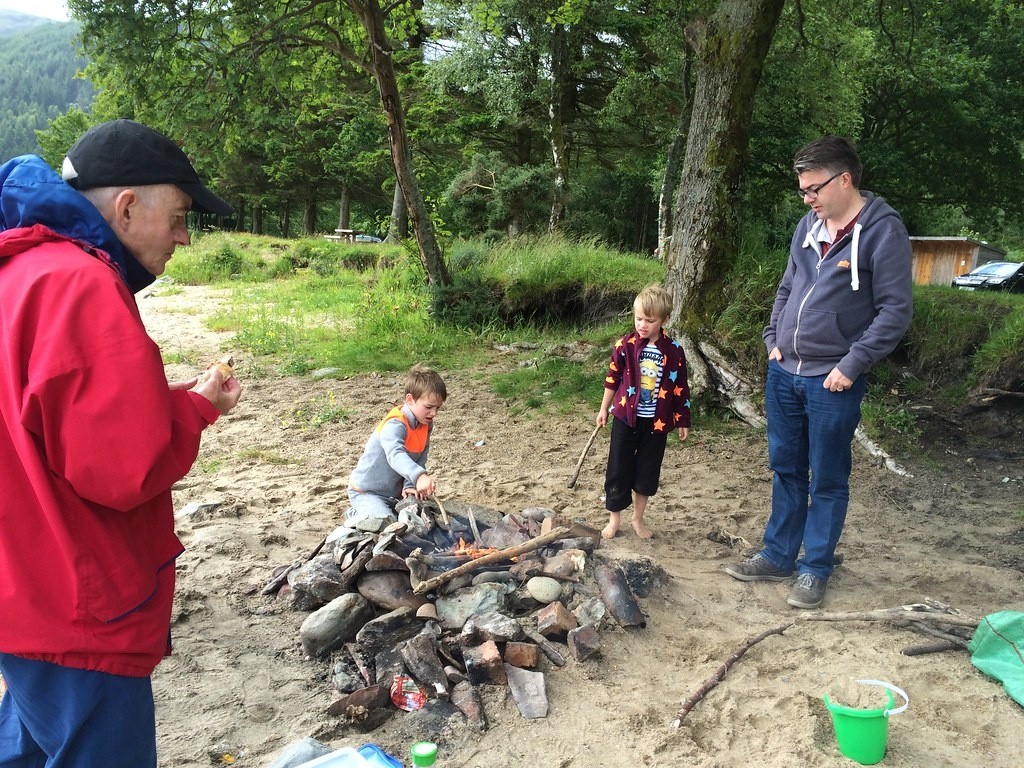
left=726, top=554, right=793, bottom=581
left=788, top=574, right=828, bottom=608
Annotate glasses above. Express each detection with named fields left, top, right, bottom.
left=798, top=172, right=844, bottom=199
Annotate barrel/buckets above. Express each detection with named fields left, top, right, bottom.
left=823, top=680, right=909, bottom=765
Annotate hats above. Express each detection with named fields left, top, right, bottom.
left=64, top=115, right=234, bottom=215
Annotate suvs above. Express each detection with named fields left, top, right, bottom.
left=951, top=260, right=1024, bottom=294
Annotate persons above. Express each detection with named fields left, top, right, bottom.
left=724, top=135, right=912, bottom=608
left=0, top=119, right=243, bottom=768
left=342, top=367, right=447, bottom=522
left=597, top=286, right=691, bottom=539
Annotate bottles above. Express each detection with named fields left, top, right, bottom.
left=411, top=741, right=438, bottom=768
